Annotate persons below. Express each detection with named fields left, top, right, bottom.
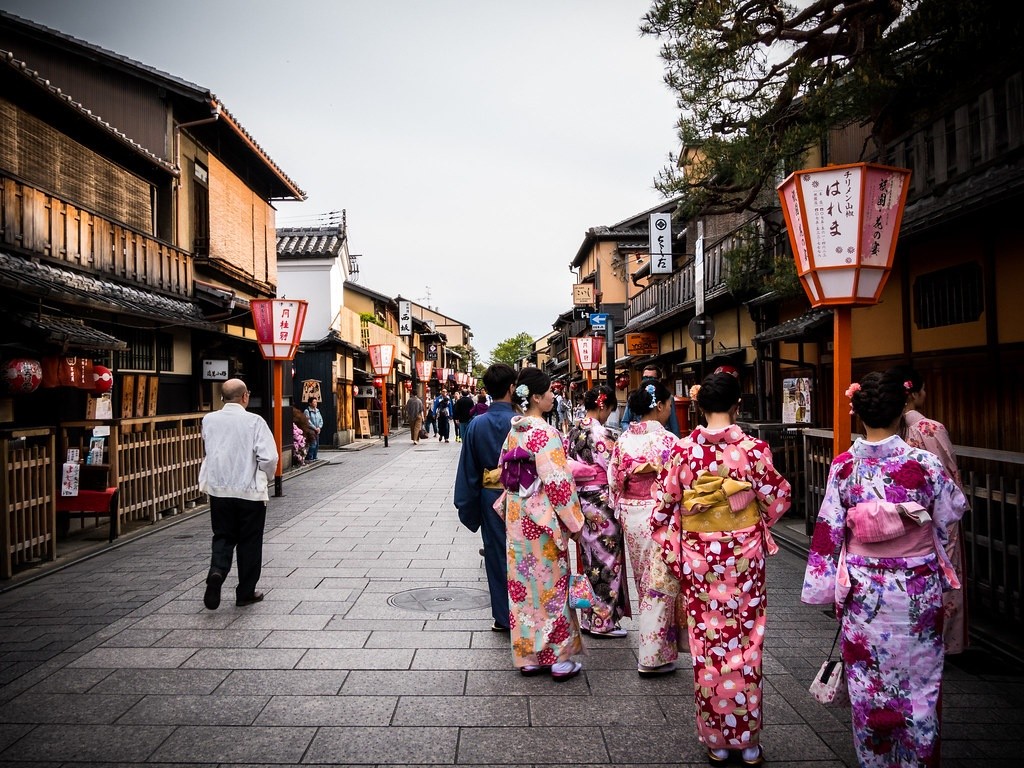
left=621, top=364, right=680, bottom=438
left=801, top=369, right=965, bottom=768
left=453, top=364, right=523, bottom=630
left=649, top=371, right=791, bottom=766
left=197, top=379, right=279, bottom=610
left=493, top=366, right=589, bottom=683
left=606, top=378, right=680, bottom=675
left=565, top=386, right=634, bottom=638
left=885, top=366, right=974, bottom=655
left=304, top=397, right=323, bottom=462
left=373, top=386, right=396, bottom=436
left=406, top=389, right=424, bottom=445
left=425, top=385, right=620, bottom=443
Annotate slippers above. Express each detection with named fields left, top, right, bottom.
left=590, top=624, right=628, bottom=639
left=491, top=621, right=504, bottom=629
left=706, top=746, right=723, bottom=760
left=552, top=660, right=582, bottom=677
left=742, top=743, right=763, bottom=764
left=637, top=660, right=675, bottom=672
left=580, top=625, right=591, bottom=634
left=520, top=666, right=552, bottom=675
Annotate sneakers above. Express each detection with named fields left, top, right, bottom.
left=203, top=573, right=222, bottom=611
left=235, top=590, right=263, bottom=606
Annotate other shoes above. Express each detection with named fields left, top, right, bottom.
left=414, top=441, right=418, bottom=444
left=445, top=439, right=449, bottom=442
left=439, top=437, right=442, bottom=441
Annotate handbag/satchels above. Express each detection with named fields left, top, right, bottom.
left=419, top=422, right=428, bottom=439
left=808, top=659, right=852, bottom=708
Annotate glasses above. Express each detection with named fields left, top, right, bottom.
left=245, top=390, right=251, bottom=398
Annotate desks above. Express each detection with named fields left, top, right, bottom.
left=52, top=483, right=118, bottom=545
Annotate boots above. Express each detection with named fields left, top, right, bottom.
left=455, top=436, right=459, bottom=441
left=459, top=438, right=461, bottom=441
